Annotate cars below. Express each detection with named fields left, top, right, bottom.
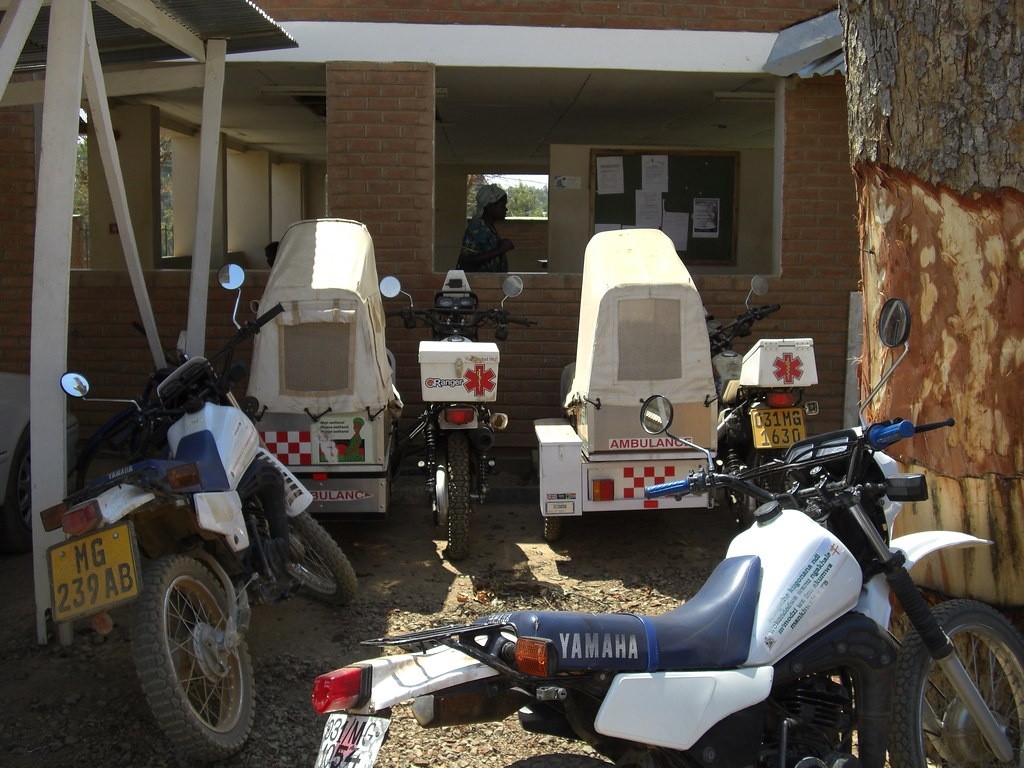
left=0, top=371, right=83, bottom=554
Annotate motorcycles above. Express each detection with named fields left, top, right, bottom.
left=310, top=298, right=1024, bottom=768
left=39, top=263, right=359, bottom=762
left=246, top=217, right=538, bottom=563
left=532, top=228, right=819, bottom=546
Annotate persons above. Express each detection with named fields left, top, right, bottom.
left=455, top=184, right=515, bottom=272
